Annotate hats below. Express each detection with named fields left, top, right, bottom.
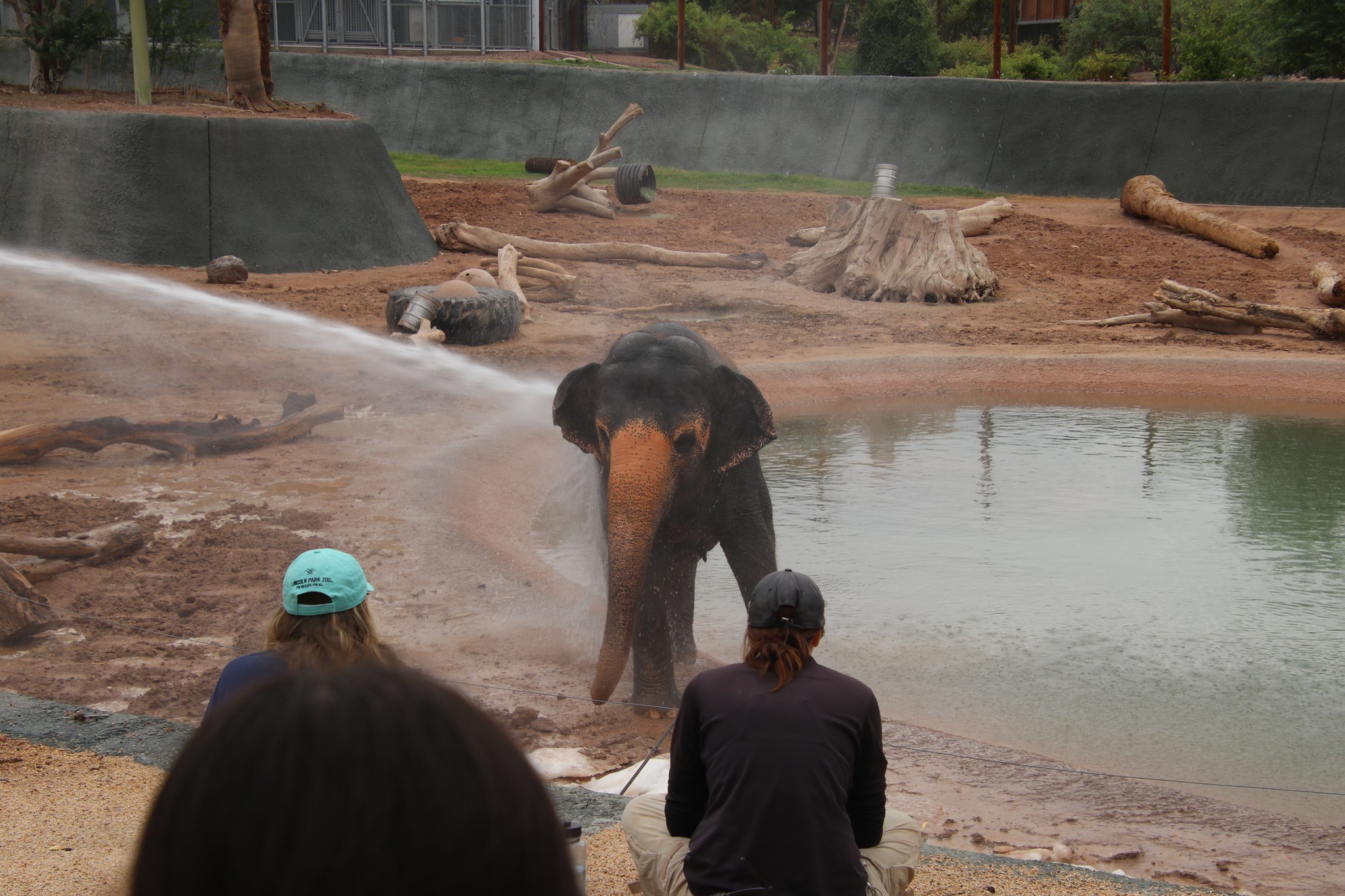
left=281, top=548, right=374, bottom=615
left=747, top=569, right=825, bottom=630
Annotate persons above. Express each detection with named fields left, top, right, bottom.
left=199, top=547, right=398, bottom=729
left=621, top=568, right=921, bottom=895
left=129, top=656, right=586, bottom=896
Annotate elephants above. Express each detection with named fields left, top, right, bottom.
left=554, top=324, right=779, bottom=718
